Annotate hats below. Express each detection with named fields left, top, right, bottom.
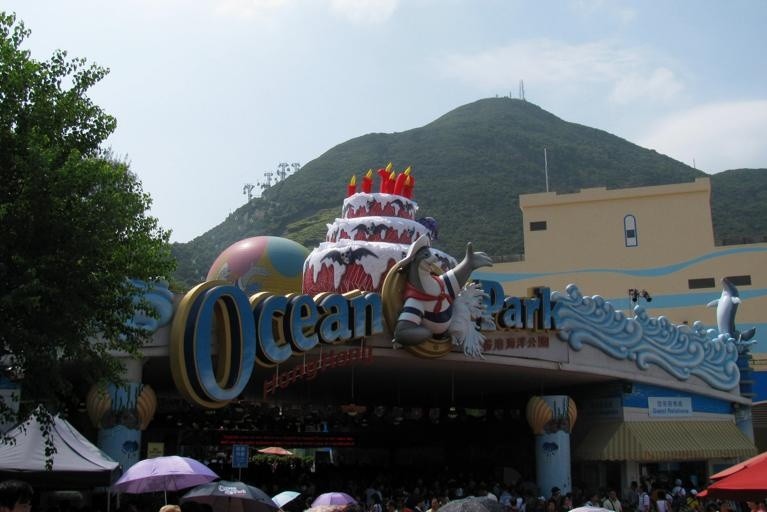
left=674, top=479, right=681, bottom=486
left=690, top=489, right=697, bottom=494
left=551, top=487, right=560, bottom=494
